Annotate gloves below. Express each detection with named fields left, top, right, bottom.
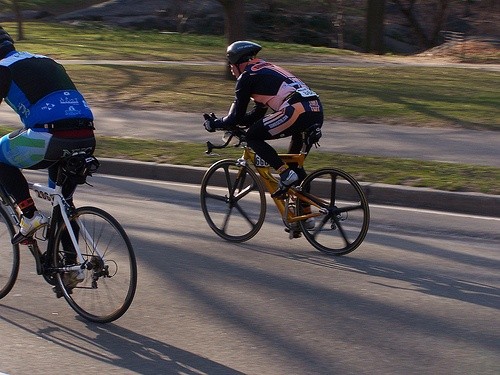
left=202, top=117, right=216, bottom=133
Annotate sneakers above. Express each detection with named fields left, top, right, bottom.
left=284, top=217, right=315, bottom=233
left=11, top=210, right=48, bottom=245
left=271, top=170, right=298, bottom=199
left=51, top=265, right=86, bottom=292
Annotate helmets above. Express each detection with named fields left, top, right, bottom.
left=0, top=24, right=14, bottom=45
left=226, top=40, right=262, bottom=64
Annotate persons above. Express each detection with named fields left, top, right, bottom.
left=203, top=41, right=324, bottom=233
left=0, top=24, right=97, bottom=294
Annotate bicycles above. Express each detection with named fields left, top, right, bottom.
left=198, top=110, right=371, bottom=257
left=0, top=151, right=138, bottom=324
